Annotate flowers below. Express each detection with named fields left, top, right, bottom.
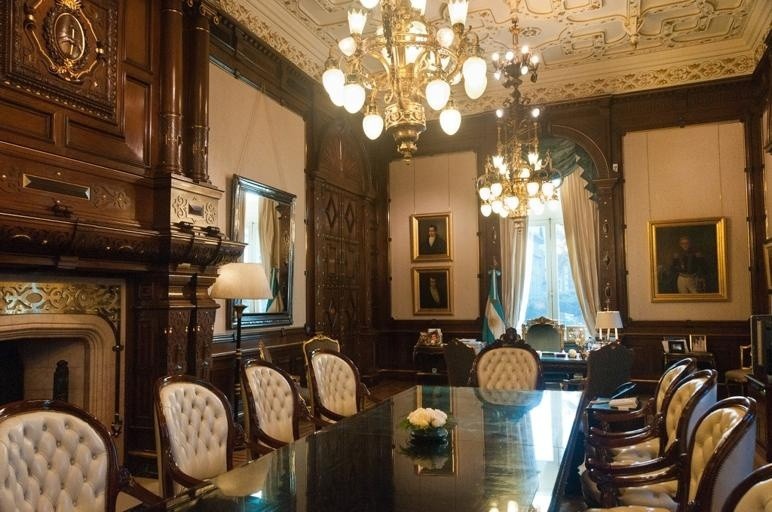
left=398, top=447, right=453, bottom=470
left=396, top=407, right=457, bottom=430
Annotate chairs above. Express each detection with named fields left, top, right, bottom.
left=304, top=350, right=381, bottom=434
left=583, top=357, right=772, bottom=512
left=303, top=333, right=341, bottom=365
left=0, top=400, right=164, bottom=512
left=441, top=317, right=567, bottom=387
left=153, top=374, right=274, bottom=499
left=239, top=357, right=334, bottom=464
left=257, top=338, right=301, bottom=386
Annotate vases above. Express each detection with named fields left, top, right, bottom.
left=410, top=429, right=448, bottom=438
left=410, top=438, right=449, bottom=447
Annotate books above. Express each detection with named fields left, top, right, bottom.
left=609, top=396, right=640, bottom=409
left=590, top=397, right=610, bottom=404
left=461, top=337, right=487, bottom=356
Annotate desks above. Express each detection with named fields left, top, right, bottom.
left=146, top=387, right=585, bottom=512
left=540, top=351, right=587, bottom=385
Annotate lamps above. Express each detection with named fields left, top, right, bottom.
left=595, top=311, right=623, bottom=340
left=211, top=264, right=274, bottom=451
left=324, top=2, right=489, bottom=164
left=475, top=17, right=563, bottom=218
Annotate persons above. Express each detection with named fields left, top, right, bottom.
left=692, top=338, right=702, bottom=352
left=424, top=275, right=447, bottom=308
left=697, top=335, right=705, bottom=351
left=425, top=225, right=446, bottom=254
left=670, top=235, right=703, bottom=293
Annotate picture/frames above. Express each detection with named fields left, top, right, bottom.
left=410, top=266, right=456, bottom=317
left=649, top=218, right=728, bottom=303
left=668, top=340, right=685, bottom=354
left=689, top=333, right=707, bottom=353
left=408, top=212, right=455, bottom=264
left=227, top=175, right=297, bottom=329
